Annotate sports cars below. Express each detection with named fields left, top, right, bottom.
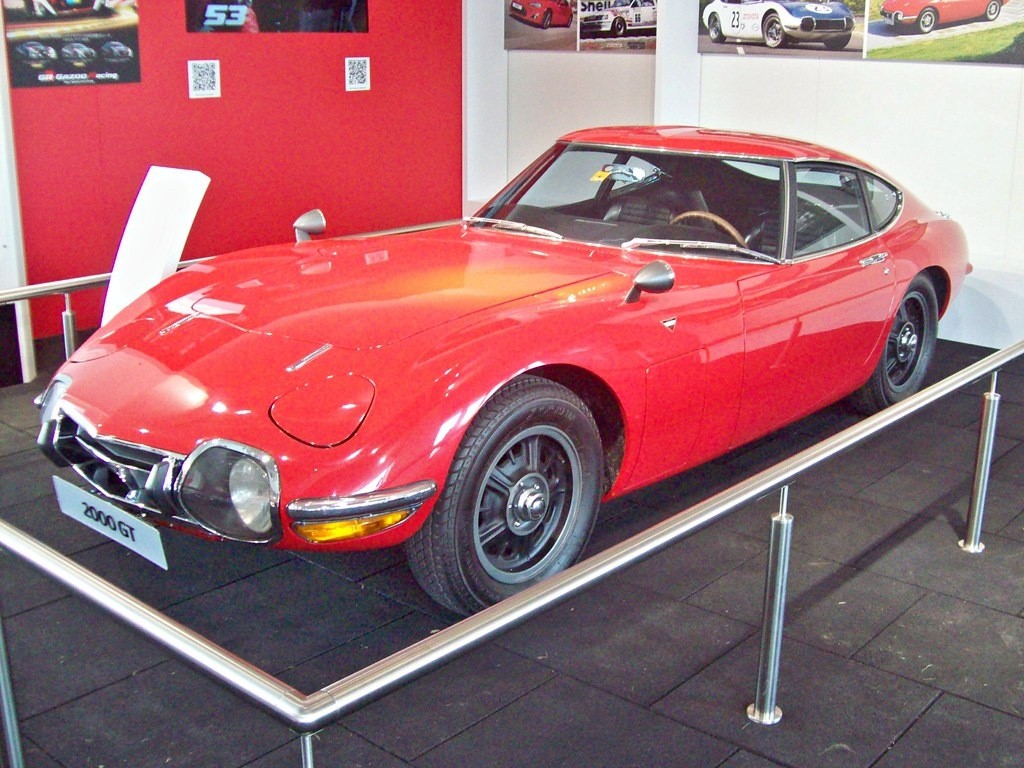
left=32, top=125, right=973, bottom=618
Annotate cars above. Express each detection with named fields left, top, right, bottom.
left=581, top=0, right=657, bottom=38
left=880, top=0, right=1009, bottom=34
left=703, top=0, right=856, bottom=51
left=510, top=0, right=573, bottom=29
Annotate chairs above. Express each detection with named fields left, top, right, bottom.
left=743, top=210, right=830, bottom=259
left=600, top=185, right=701, bottom=228
